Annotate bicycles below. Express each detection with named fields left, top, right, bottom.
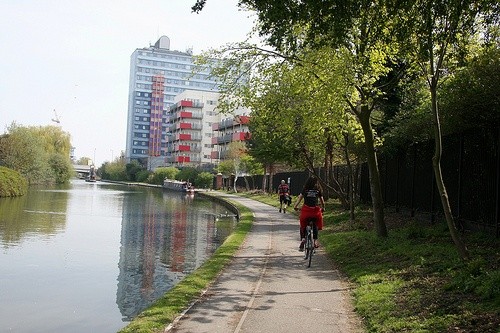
left=295, top=207, right=325, bottom=267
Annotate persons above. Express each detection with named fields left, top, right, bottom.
left=294, top=174, right=324, bottom=249
left=278, top=180, right=290, bottom=213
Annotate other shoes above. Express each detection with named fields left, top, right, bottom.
left=284, top=210, right=286, bottom=213
left=314, top=239, right=319, bottom=248
left=299, top=238, right=306, bottom=250
left=279, top=210, right=282, bottom=213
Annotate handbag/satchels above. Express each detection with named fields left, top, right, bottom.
left=285, top=196, right=290, bottom=200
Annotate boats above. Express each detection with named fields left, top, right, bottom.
left=163, top=179, right=194, bottom=193
